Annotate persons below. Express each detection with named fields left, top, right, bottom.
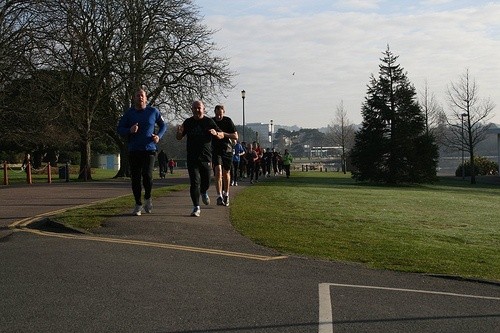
left=158, top=150, right=176, bottom=179
left=212, top=138, right=293, bottom=194
left=118, top=89, right=167, bottom=216
left=211, top=105, right=238, bottom=206
left=176, top=100, right=224, bottom=216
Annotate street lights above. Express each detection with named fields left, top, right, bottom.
left=241, top=89, right=246, bottom=143
left=270, top=120, right=274, bottom=149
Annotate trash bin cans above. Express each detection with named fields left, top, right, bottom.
left=58, top=166, right=68, bottom=182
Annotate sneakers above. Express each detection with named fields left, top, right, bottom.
left=133, top=204, right=142, bottom=216
left=143, top=195, right=152, bottom=213
left=191, top=205, right=200, bottom=217
left=216, top=196, right=225, bottom=206
left=223, top=194, right=229, bottom=206
left=199, top=190, right=210, bottom=205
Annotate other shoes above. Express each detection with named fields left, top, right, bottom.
left=230, top=172, right=277, bottom=186
